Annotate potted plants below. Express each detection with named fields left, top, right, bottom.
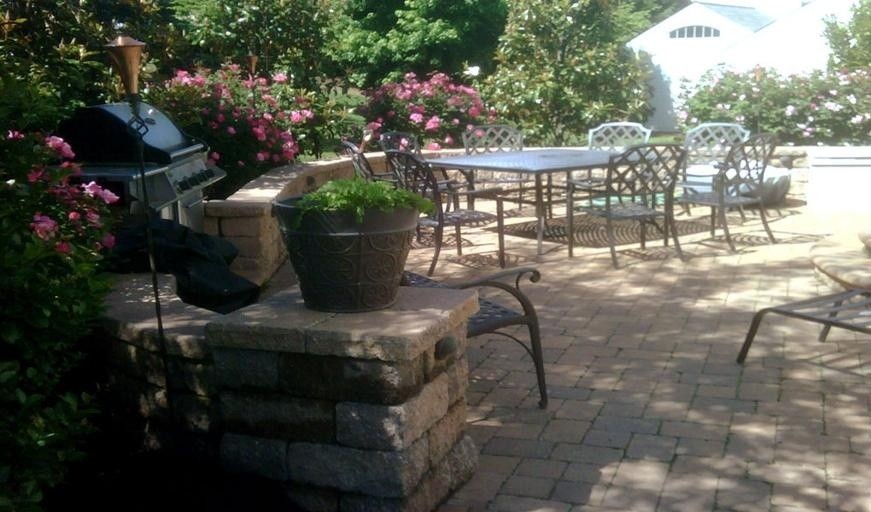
left=271, top=173, right=438, bottom=314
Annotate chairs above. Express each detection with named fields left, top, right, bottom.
left=343, top=124, right=779, bottom=275
left=399, top=264, right=550, bottom=407
